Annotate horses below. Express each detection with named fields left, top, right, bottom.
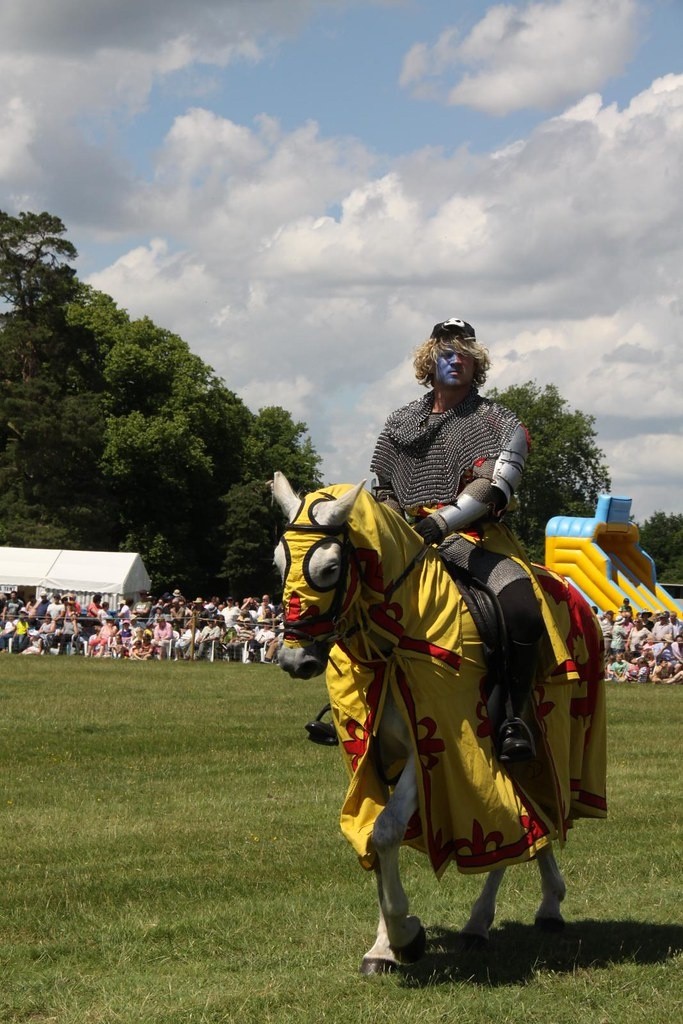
left=271, top=468, right=606, bottom=971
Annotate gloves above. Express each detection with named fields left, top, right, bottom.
left=414, top=517, right=443, bottom=544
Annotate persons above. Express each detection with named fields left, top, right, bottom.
left=592, top=598, right=683, bottom=684
left=0, top=591, right=285, bottom=665
left=304, top=317, right=544, bottom=757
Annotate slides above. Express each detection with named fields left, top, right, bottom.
left=544, top=515, right=683, bottom=622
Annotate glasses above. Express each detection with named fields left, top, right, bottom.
left=140, top=593, right=146, bottom=595
left=228, top=599, right=232, bottom=602
left=634, top=621, right=640, bottom=623
left=54, top=596, right=59, bottom=599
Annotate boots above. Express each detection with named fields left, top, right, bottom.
left=305, top=720, right=336, bottom=738
left=501, top=635, right=543, bottom=756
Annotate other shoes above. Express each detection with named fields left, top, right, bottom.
left=174, top=658, right=180, bottom=662
left=95, top=653, right=102, bottom=657
left=113, top=656, right=120, bottom=660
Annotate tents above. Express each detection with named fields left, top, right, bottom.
left=0, top=546, right=151, bottom=612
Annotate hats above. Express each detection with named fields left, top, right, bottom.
left=640, top=608, right=652, bottom=618
left=118, top=600, right=125, bottom=604
left=122, top=620, right=130, bottom=625
left=192, top=597, right=204, bottom=603
left=615, top=616, right=625, bottom=624
left=18, top=607, right=29, bottom=615
left=173, top=589, right=181, bottom=596
left=663, top=634, right=674, bottom=642
left=606, top=610, right=616, bottom=615
left=430, top=318, right=476, bottom=341
